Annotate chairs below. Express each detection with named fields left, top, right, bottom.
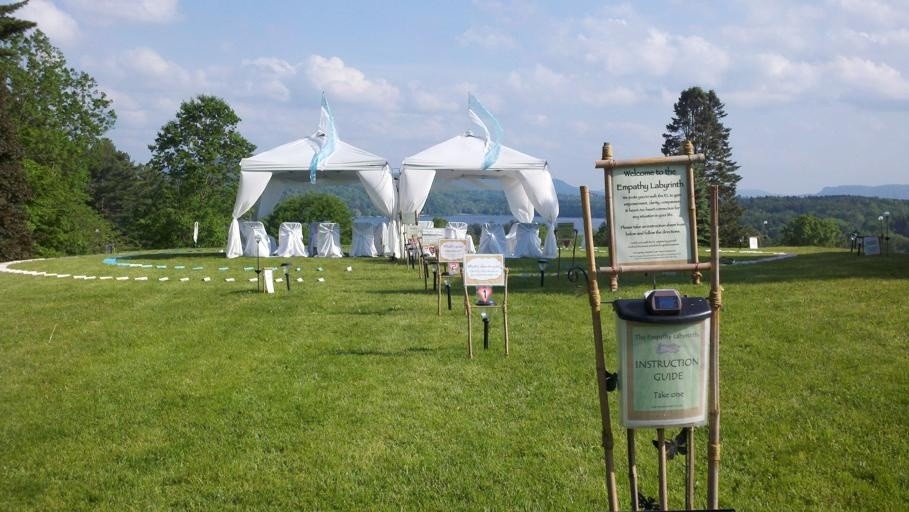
left=316, top=223, right=345, bottom=257
left=445, top=222, right=468, bottom=239
left=505, top=223, right=518, bottom=255
left=374, top=217, right=390, bottom=257
left=308, top=222, right=317, bottom=256
left=465, top=234, right=476, bottom=255
left=268, top=234, right=277, bottom=252
left=514, top=222, right=543, bottom=257
left=239, top=221, right=271, bottom=257
left=349, top=216, right=377, bottom=258
left=418, top=221, right=434, bottom=227
left=274, top=223, right=311, bottom=258
left=479, top=223, right=507, bottom=254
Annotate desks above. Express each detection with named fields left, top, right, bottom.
left=422, top=228, right=445, bottom=258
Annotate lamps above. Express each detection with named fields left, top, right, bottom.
left=281, top=262, right=293, bottom=290
left=475, top=301, right=498, bottom=351
left=537, top=259, right=548, bottom=287
left=409, top=246, right=416, bottom=270
left=428, top=260, right=438, bottom=293
left=439, top=272, right=454, bottom=309
left=421, top=254, right=431, bottom=278
left=847, top=230, right=863, bottom=255
